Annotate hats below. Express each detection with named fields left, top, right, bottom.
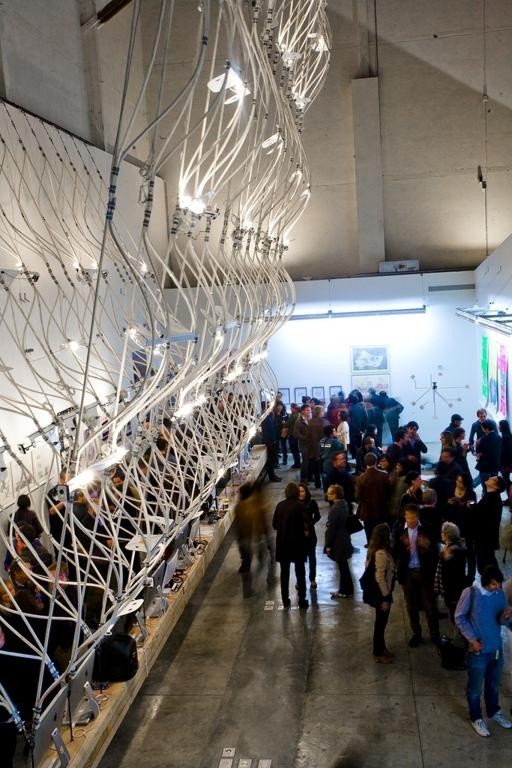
left=450, top=415, right=464, bottom=420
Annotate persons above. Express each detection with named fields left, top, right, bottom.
left=392, top=504, right=439, bottom=648
left=261, top=391, right=354, bottom=506
left=454, top=566, right=512, bottom=736
left=323, top=484, right=354, bottom=598
left=272, top=482, right=321, bottom=610
left=429, top=408, right=512, bottom=599
left=351, top=387, right=443, bottom=548
left=364, top=522, right=398, bottom=664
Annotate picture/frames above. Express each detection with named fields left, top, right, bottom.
left=350, top=373, right=391, bottom=404
left=329, top=386, right=342, bottom=402
left=295, top=387, right=308, bottom=404
left=261, top=389, right=274, bottom=403
left=312, top=386, right=326, bottom=403
left=350, top=345, right=390, bottom=372
left=278, top=388, right=290, bottom=404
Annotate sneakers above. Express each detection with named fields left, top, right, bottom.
left=375, top=653, right=394, bottom=664
left=470, top=718, right=492, bottom=737
left=492, top=710, right=511, bottom=729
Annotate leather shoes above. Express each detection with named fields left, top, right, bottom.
left=266, top=475, right=282, bottom=483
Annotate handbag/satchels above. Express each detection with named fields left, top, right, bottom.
left=359, top=549, right=387, bottom=608
left=453, top=586, right=474, bottom=646
left=348, top=510, right=363, bottom=536
